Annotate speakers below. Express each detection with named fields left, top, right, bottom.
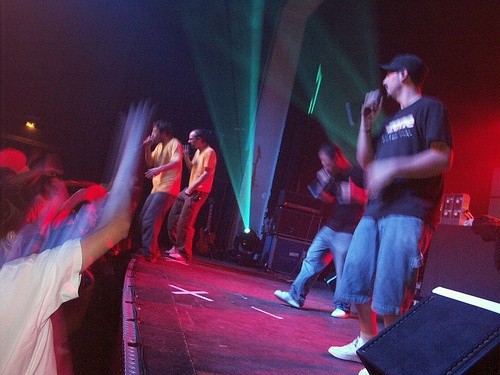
left=356, top=285, right=500, bottom=375
left=268, top=205, right=323, bottom=242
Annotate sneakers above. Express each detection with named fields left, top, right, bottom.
left=358, top=368, right=370, bottom=375
left=274, top=290, right=300, bottom=308
left=328, top=336, right=364, bottom=362
left=331, top=309, right=346, bottom=317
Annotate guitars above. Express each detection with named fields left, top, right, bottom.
left=195, top=197, right=217, bottom=257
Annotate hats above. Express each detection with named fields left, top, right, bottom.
left=378, top=56, right=424, bottom=79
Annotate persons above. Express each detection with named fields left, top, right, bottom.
left=274, top=143, right=368, bottom=320
left=133, top=119, right=183, bottom=258
left=328, top=56, right=452, bottom=375
left=0, top=103, right=153, bottom=375
left=166, top=129, right=218, bottom=262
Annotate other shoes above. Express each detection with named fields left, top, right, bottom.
left=165, top=246, right=180, bottom=254
left=169, top=254, right=186, bottom=261
